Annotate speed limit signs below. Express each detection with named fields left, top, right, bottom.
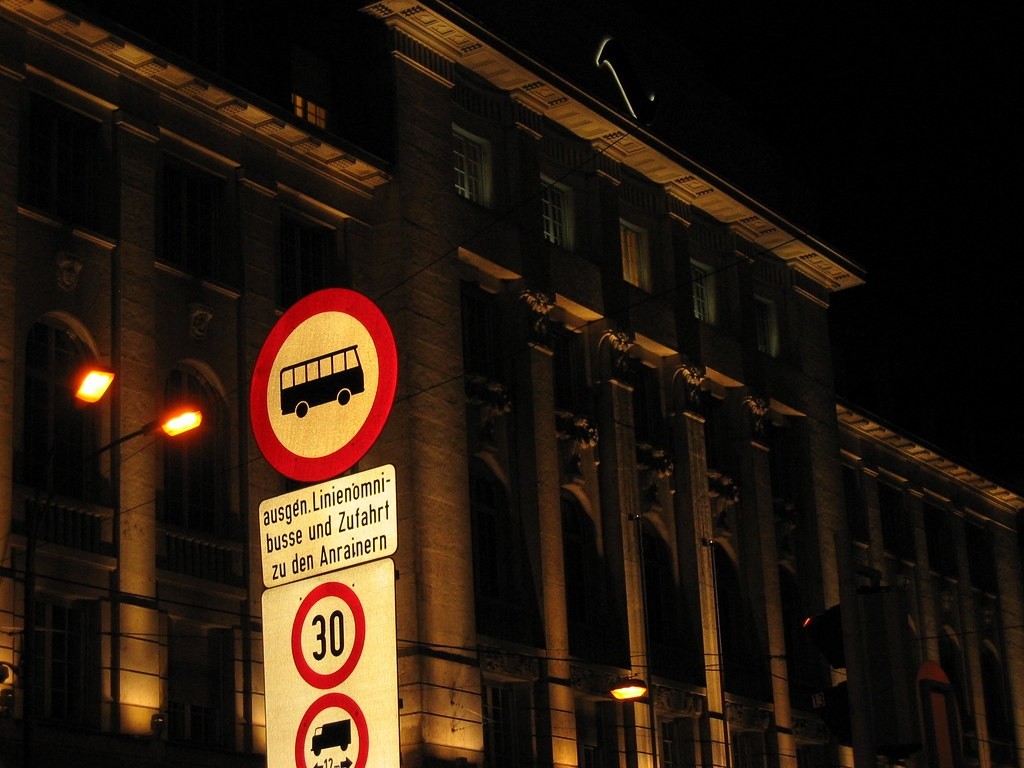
left=292, top=582, right=365, bottom=689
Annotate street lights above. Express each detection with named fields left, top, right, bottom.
left=23, top=365, right=203, bottom=768
